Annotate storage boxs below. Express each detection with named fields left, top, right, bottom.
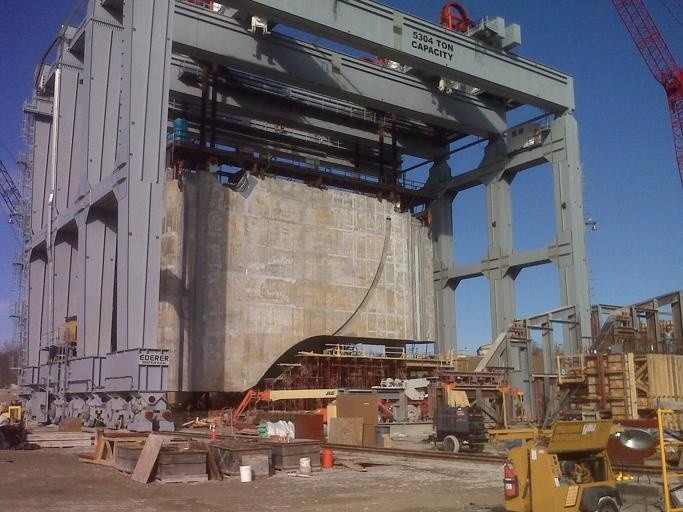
left=363, top=424, right=376, bottom=446
left=336, top=393, right=378, bottom=424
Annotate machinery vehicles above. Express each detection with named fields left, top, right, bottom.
left=492, top=411, right=679, bottom=511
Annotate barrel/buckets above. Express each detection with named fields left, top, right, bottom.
left=323, top=449, right=333, bottom=469
left=240, top=465, right=252, bottom=482
left=299, top=457, right=312, bottom=474
